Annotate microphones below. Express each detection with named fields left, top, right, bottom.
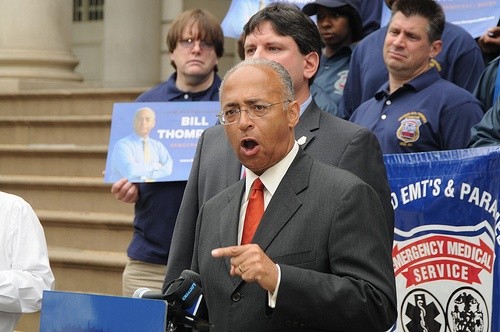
left=165, top=269, right=202, bottom=332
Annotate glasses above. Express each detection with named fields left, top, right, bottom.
left=216, top=99, right=293, bottom=125
left=179, top=38, right=215, bottom=51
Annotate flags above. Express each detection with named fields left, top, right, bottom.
left=383, top=146, right=500, bottom=332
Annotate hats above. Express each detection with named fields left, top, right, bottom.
left=302, top=0, right=362, bottom=28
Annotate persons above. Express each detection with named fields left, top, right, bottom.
left=0, top=191, right=56, bottom=332
left=302, top=0, right=362, bottom=114
left=190, top=59, right=398, bottom=332
left=101, top=8, right=222, bottom=300
left=338, top=0, right=486, bottom=120
left=463, top=94, right=500, bottom=149
left=161, top=2, right=394, bottom=332
left=472, top=55, right=500, bottom=111
left=347, top=0, right=485, bottom=155
left=478, top=27, right=500, bottom=59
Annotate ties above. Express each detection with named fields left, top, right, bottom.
left=241, top=179, right=265, bottom=245
